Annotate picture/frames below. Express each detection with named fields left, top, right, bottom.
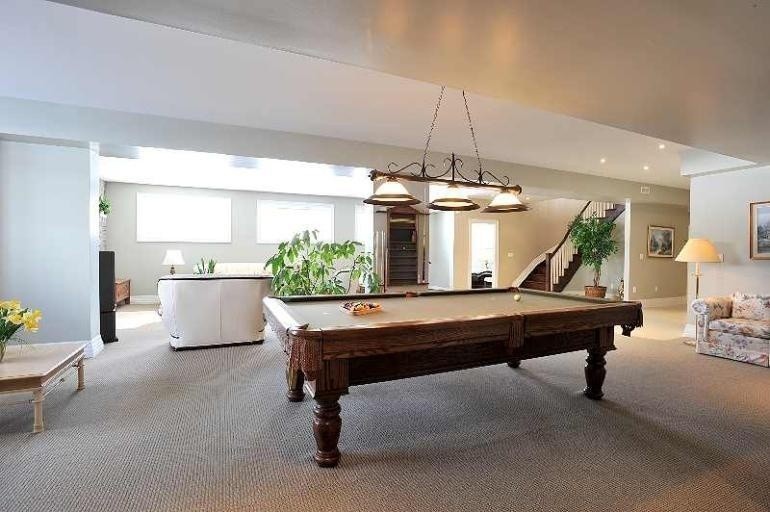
left=747, top=200, right=770, bottom=260
left=646, top=224, right=676, bottom=259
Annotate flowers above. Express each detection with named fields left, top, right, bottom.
left=0, top=298, right=43, bottom=360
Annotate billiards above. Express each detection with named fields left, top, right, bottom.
left=339, top=299, right=382, bottom=315
left=514, top=293, right=523, bottom=302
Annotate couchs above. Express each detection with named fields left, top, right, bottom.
left=691, top=289, right=770, bottom=370
left=156, top=272, right=275, bottom=353
left=193, top=262, right=280, bottom=275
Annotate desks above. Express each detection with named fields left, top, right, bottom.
left=115, top=276, right=132, bottom=308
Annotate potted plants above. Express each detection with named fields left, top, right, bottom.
left=566, top=211, right=620, bottom=299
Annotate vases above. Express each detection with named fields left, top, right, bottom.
left=0, top=342, right=7, bottom=363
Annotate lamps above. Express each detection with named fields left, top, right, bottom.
left=160, top=249, right=185, bottom=275
left=674, top=239, right=722, bottom=347
left=483, top=259, right=490, bottom=270
left=361, top=86, right=532, bottom=215
